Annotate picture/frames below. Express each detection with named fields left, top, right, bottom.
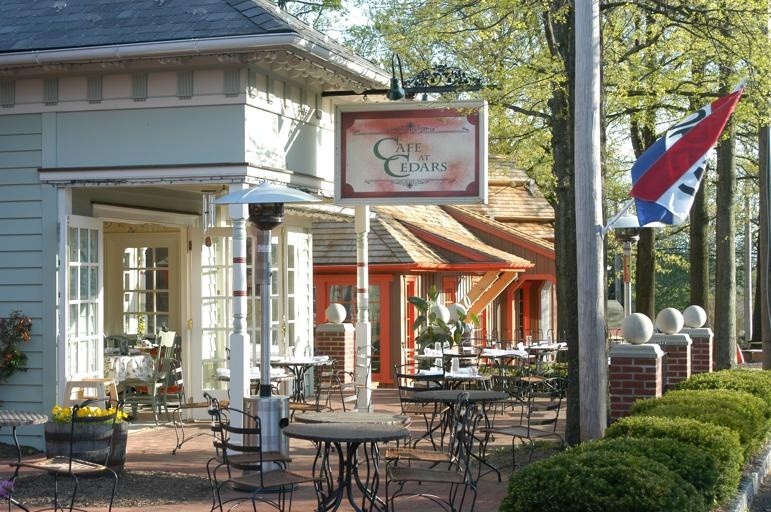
left=334, top=99, right=488, bottom=206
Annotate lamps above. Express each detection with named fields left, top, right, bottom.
left=379, top=50, right=411, bottom=102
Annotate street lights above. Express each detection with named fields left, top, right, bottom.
left=615, top=222, right=640, bottom=320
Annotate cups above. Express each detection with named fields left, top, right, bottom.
left=495, top=342, right=513, bottom=354
left=435, top=358, right=443, bottom=372
left=287, top=344, right=312, bottom=361
left=451, top=357, right=459, bottom=375
left=526, top=335, right=532, bottom=347
left=434, top=340, right=450, bottom=356
left=106, top=338, right=129, bottom=355
left=470, top=368, right=478, bottom=377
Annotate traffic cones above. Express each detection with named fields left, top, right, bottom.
left=735, top=343, right=744, bottom=365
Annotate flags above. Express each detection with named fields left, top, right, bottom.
left=628, top=87, right=739, bottom=227
